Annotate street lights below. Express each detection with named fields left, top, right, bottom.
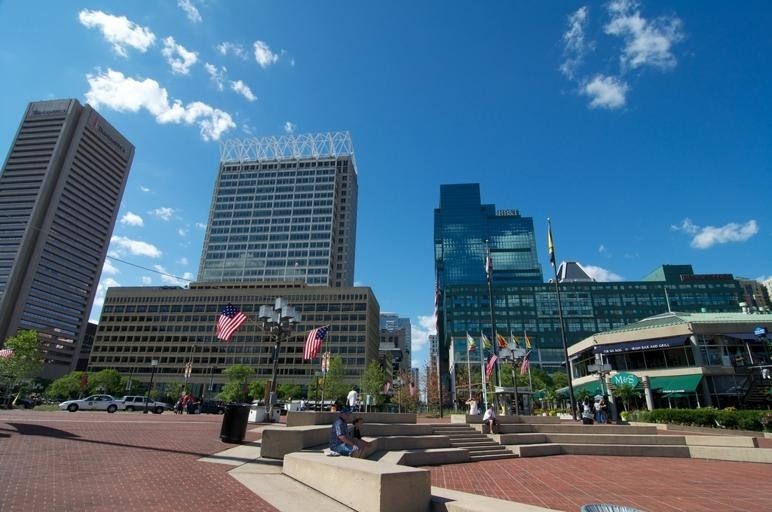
left=587, top=358, right=613, bottom=396
left=497, top=340, right=528, bottom=415
left=142, top=358, right=158, bottom=413
left=259, top=296, right=305, bottom=411
left=314, top=371, right=322, bottom=412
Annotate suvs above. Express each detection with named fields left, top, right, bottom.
left=120, top=395, right=170, bottom=415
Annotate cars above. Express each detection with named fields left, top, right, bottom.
left=0, top=392, right=63, bottom=410
left=189, top=398, right=253, bottom=416
left=58, top=394, right=127, bottom=414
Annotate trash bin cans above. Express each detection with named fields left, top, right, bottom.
left=219, top=402, right=252, bottom=444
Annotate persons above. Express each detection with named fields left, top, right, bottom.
left=346, top=385, right=359, bottom=412
left=599, top=395, right=608, bottom=424
left=349, top=418, right=373, bottom=449
left=582, top=397, right=591, bottom=414
left=465, top=397, right=480, bottom=416
left=173, top=397, right=193, bottom=415
left=482, top=406, right=500, bottom=435
left=329, top=407, right=365, bottom=458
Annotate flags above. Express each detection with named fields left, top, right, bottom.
left=486, top=244, right=493, bottom=289
left=547, top=220, right=554, bottom=268
left=467, top=332, right=532, bottom=351
left=215, top=303, right=247, bottom=341
left=303, top=326, right=329, bottom=360
left=520, top=351, right=533, bottom=376
left=485, top=353, right=497, bottom=381
